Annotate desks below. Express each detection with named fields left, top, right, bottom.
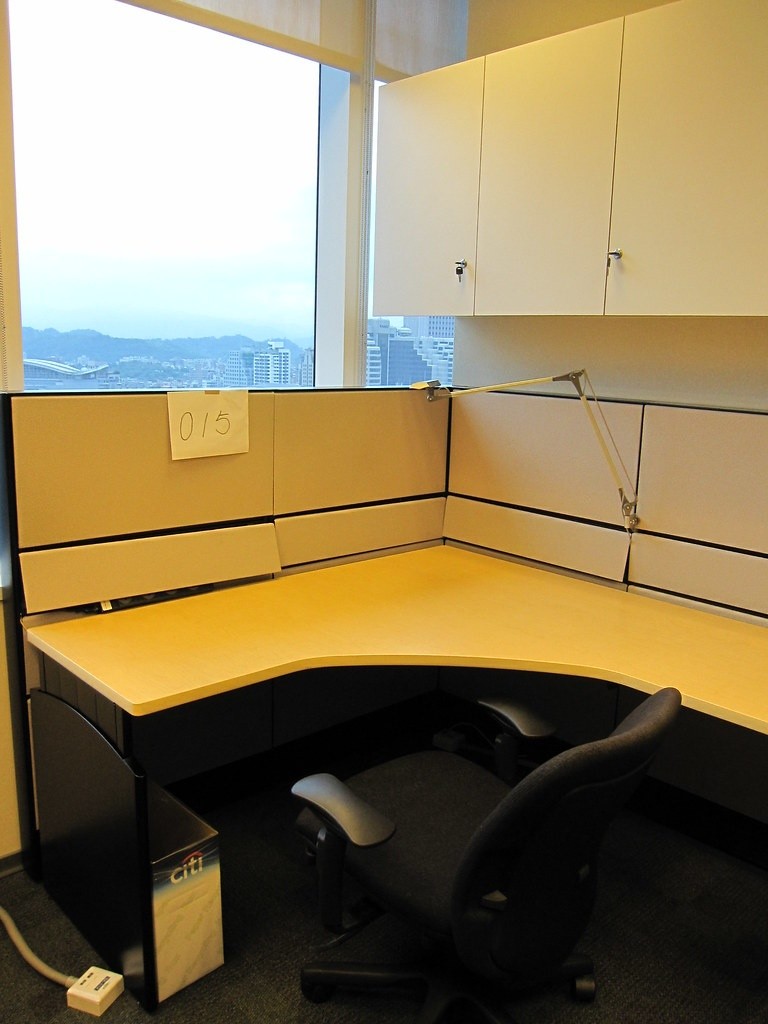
left=29, top=545, right=767, bottom=1015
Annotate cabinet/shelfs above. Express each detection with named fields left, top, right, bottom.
left=605, top=0, right=768, bottom=317
left=371, top=15, right=624, bottom=318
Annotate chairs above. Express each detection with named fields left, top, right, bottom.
left=290, top=686, right=681, bottom=1024
